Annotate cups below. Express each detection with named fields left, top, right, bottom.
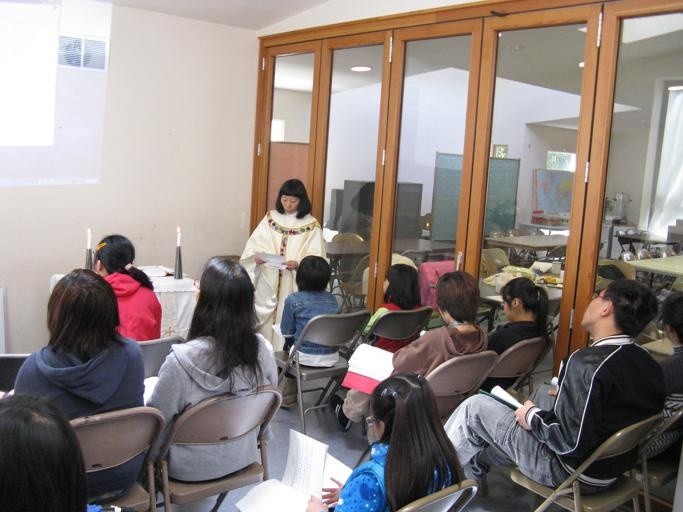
left=552, top=261, right=562, bottom=278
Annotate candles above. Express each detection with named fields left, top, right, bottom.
left=86, top=227, right=92, bottom=249
left=176, top=224, right=182, bottom=247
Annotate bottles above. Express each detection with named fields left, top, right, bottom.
left=495, top=273, right=502, bottom=293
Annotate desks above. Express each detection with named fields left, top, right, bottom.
left=324, top=237, right=457, bottom=309
left=626, top=254, right=683, bottom=290
left=485, top=232, right=568, bottom=261
left=611, top=232, right=680, bottom=261
left=477, top=266, right=614, bottom=333
left=49, top=265, right=201, bottom=337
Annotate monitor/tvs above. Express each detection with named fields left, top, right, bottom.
left=342, top=179, right=425, bottom=239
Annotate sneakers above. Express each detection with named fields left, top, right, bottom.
left=328, top=394, right=352, bottom=433
left=281, top=395, right=297, bottom=410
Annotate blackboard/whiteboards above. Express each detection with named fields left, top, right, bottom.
left=429, top=153, right=520, bottom=244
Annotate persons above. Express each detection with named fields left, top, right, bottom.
left=636, top=291, right=683, bottom=460
left=0, top=395, right=86, bottom=512
left=329, top=271, right=489, bottom=432
left=146, top=256, right=279, bottom=483
left=304, top=372, right=466, bottom=512
left=14, top=268, right=145, bottom=502
left=479, top=276, right=553, bottom=392
left=93, top=234, right=162, bottom=342
left=239, top=179, right=326, bottom=352
left=337, top=182, right=374, bottom=281
left=362, top=263, right=421, bottom=352
left=443, top=278, right=667, bottom=496
left=278, top=255, right=339, bottom=408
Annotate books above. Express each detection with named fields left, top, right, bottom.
left=341, top=344, right=393, bottom=395
left=235, top=426, right=353, bottom=512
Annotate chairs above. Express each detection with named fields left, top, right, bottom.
left=627, top=410, right=683, bottom=512
left=154, top=386, right=283, bottom=512
left=270, top=309, right=373, bottom=435
left=335, top=252, right=372, bottom=312
left=481, top=246, right=507, bottom=280
left=598, top=258, right=637, bottom=282
left=1, top=353, right=34, bottom=392
left=320, top=305, right=434, bottom=412
left=133, top=334, right=185, bottom=381
left=399, top=250, right=429, bottom=267
left=425, top=247, right=456, bottom=260
left=510, top=413, right=664, bottom=512
left=67, top=405, right=166, bottom=512
left=419, top=260, right=455, bottom=306
left=331, top=232, right=365, bottom=243
left=395, top=479, right=480, bottom=512
left=425, top=350, right=499, bottom=416
left=495, top=335, right=548, bottom=400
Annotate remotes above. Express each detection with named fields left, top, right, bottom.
left=356, top=232, right=366, bottom=241
